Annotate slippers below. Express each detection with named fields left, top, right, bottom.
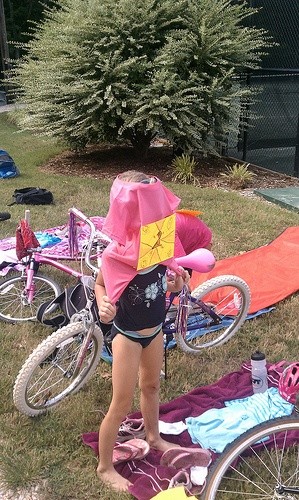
left=160, top=447, right=211, bottom=469
left=112, top=438, right=150, bottom=466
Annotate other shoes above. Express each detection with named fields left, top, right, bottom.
left=0, top=213, right=11, bottom=221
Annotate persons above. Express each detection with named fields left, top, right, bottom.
left=166, top=212, right=213, bottom=299
left=94, top=170, right=190, bottom=492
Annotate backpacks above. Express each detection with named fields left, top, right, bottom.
left=7, top=187, right=53, bottom=206
left=0, top=149, right=19, bottom=178
left=36, top=283, right=114, bottom=339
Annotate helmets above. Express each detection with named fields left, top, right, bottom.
left=278, top=362, right=299, bottom=404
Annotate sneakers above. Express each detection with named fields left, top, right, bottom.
left=166, top=470, right=206, bottom=497
left=115, top=410, right=146, bottom=442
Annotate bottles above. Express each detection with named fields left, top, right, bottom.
left=251, top=349, right=268, bottom=394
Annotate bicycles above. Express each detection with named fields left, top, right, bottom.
left=0, top=210, right=104, bottom=326
left=12, top=207, right=255, bottom=413
